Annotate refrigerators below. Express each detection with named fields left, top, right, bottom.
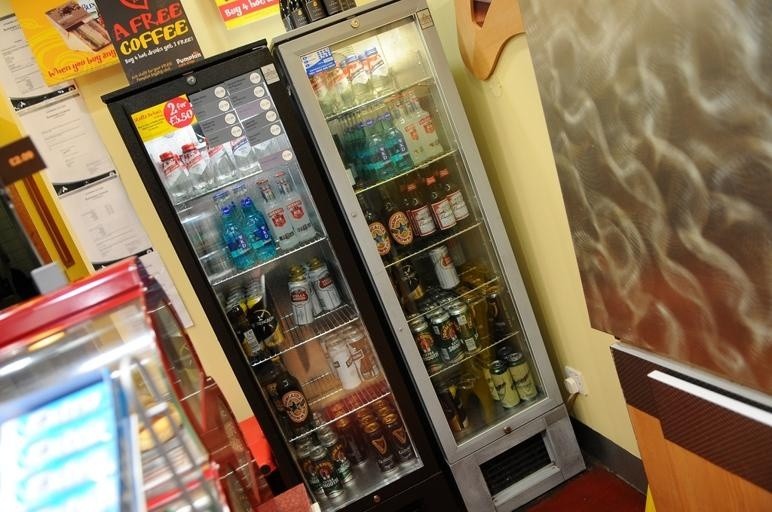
left=102, top=42, right=462, bottom=511
left=274, top=0, right=587, bottom=511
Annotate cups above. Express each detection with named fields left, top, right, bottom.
left=31, top=264, right=70, bottom=294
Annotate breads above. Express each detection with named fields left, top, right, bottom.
left=132, top=397, right=184, bottom=455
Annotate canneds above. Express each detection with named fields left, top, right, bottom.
left=225, top=256, right=419, bottom=499
left=387, top=242, right=540, bottom=434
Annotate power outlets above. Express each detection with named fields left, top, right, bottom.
left=563, top=365, right=588, bottom=397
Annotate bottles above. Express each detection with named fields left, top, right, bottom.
left=310, top=49, right=474, bottom=265
left=147, top=126, right=317, bottom=274
left=279, top=0, right=355, bottom=31
